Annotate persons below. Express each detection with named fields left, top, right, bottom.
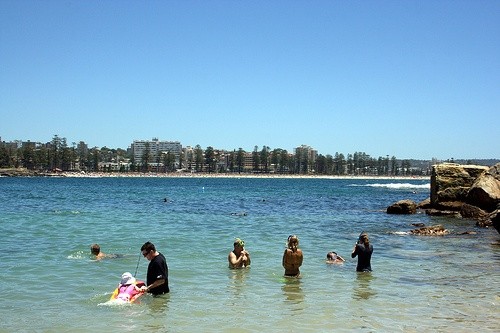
left=140, top=241, right=170, bottom=298
left=326, top=251, right=344, bottom=264
left=228, top=238, right=251, bottom=269
left=89, top=244, right=104, bottom=263
left=282, top=235, right=304, bottom=278
left=115, top=272, right=150, bottom=304
left=351, top=231, right=373, bottom=272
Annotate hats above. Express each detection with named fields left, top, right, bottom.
left=121, top=272, right=136, bottom=285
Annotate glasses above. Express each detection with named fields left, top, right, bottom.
left=237, top=240, right=245, bottom=248
left=143, top=252, right=150, bottom=257
